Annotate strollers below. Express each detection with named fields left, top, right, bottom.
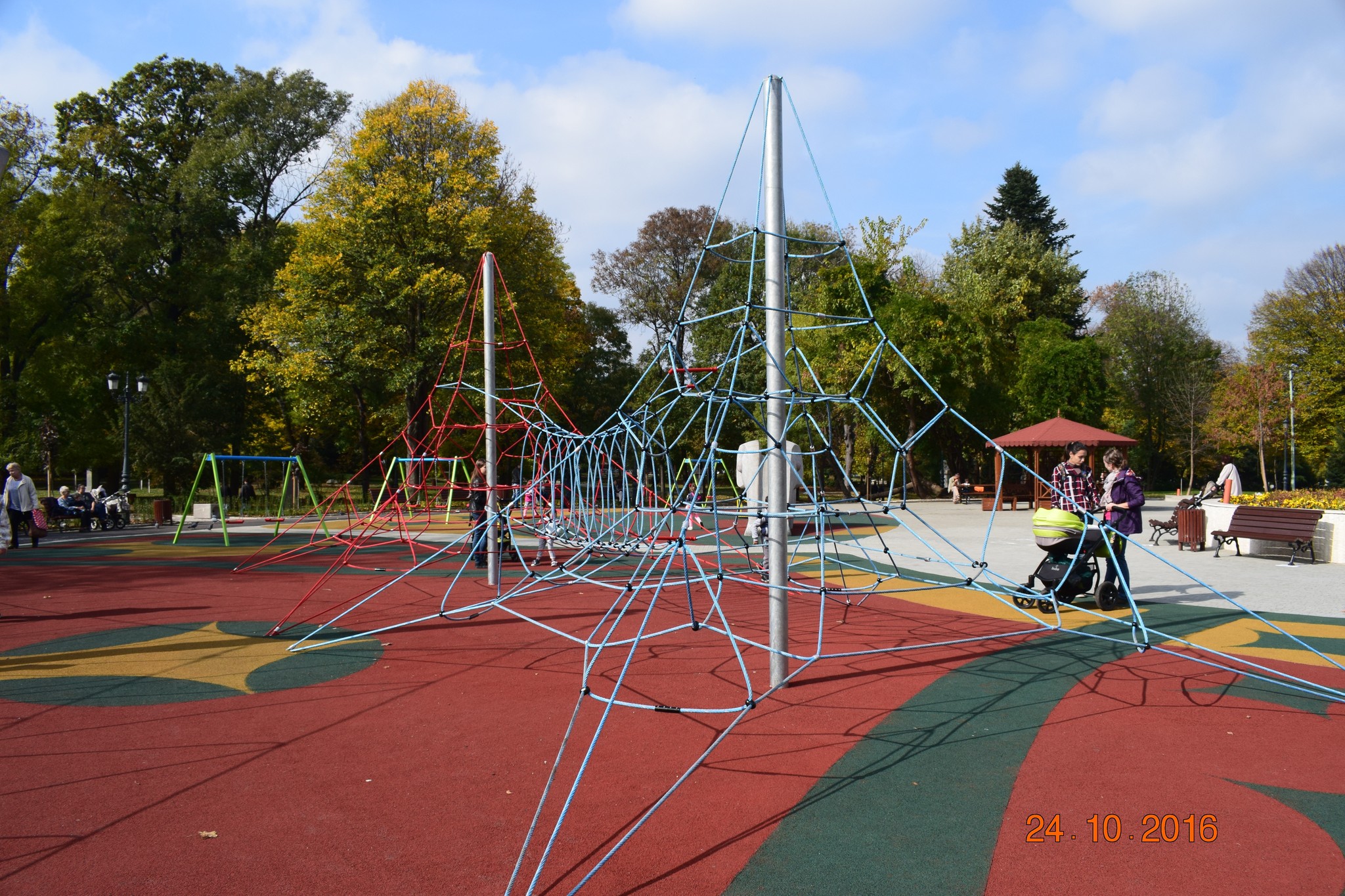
left=1011, top=504, right=1127, bottom=615
left=469, top=496, right=519, bottom=563
left=90, top=485, right=131, bottom=530
left=1191, top=481, right=1222, bottom=509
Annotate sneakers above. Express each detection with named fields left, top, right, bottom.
left=551, top=560, right=557, bottom=566
left=514, top=506, right=521, bottom=509
left=531, top=559, right=540, bottom=566
left=687, top=527, right=693, bottom=530
left=475, top=561, right=488, bottom=568
left=761, top=573, right=769, bottom=582
left=756, top=562, right=763, bottom=576
left=699, top=521, right=704, bottom=529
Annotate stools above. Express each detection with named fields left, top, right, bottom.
left=1038, top=498, right=1052, bottom=511
left=982, top=498, right=999, bottom=512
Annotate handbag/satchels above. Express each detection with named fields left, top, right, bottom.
left=28, top=517, right=47, bottom=538
left=1221, top=484, right=1225, bottom=492
left=31, top=509, right=48, bottom=530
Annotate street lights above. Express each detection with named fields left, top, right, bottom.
left=106, top=369, right=151, bottom=526
left=880, top=453, right=886, bottom=500
left=1282, top=418, right=1289, bottom=491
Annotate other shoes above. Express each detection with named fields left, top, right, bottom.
left=958, top=498, right=960, bottom=501
left=8, top=544, right=18, bottom=549
left=32, top=544, right=38, bottom=548
left=1117, top=597, right=1129, bottom=606
left=954, top=501, right=958, bottom=504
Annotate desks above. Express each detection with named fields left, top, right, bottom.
left=956, top=484, right=995, bottom=504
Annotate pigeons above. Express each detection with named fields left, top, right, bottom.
left=205, top=523, right=214, bottom=531
left=187, top=521, right=200, bottom=528
left=155, top=520, right=159, bottom=528
left=339, top=510, right=346, bottom=516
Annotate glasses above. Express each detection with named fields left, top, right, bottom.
left=79, top=488, right=86, bottom=490
left=64, top=491, right=70, bottom=493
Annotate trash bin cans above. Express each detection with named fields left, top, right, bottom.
left=496, top=487, right=507, bottom=499
left=1178, top=508, right=1206, bottom=543
left=154, top=499, right=172, bottom=526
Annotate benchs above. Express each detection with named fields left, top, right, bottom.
left=1148, top=499, right=1195, bottom=546
left=1001, top=485, right=1034, bottom=511
left=39, top=497, right=107, bottom=533
left=440, top=489, right=470, bottom=511
left=369, top=488, right=418, bottom=510
left=1210, top=505, right=1324, bottom=566
left=538, top=486, right=567, bottom=508
left=962, top=491, right=995, bottom=495
left=592, top=487, right=632, bottom=507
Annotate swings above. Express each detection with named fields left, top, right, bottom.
left=434, top=461, right=451, bottom=508
left=691, top=462, right=701, bottom=484
left=264, top=462, right=284, bottom=522
left=705, top=462, right=716, bottom=498
left=222, top=461, right=245, bottom=524
left=405, top=462, right=421, bottom=508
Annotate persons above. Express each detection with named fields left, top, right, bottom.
left=1051, top=442, right=1099, bottom=597
left=512, top=462, right=525, bottom=509
left=614, top=479, right=623, bottom=505
left=3, top=463, right=38, bottom=549
left=469, top=458, right=488, bottom=568
left=752, top=496, right=769, bottom=582
left=524, top=481, right=548, bottom=516
left=686, top=483, right=704, bottom=530
left=530, top=509, right=561, bottom=566
left=1213, top=456, right=1242, bottom=495
left=236, top=477, right=254, bottom=514
left=58, top=484, right=108, bottom=532
left=948, top=473, right=970, bottom=504
left=1093, top=449, right=1145, bottom=602
left=860, top=481, right=884, bottom=501
